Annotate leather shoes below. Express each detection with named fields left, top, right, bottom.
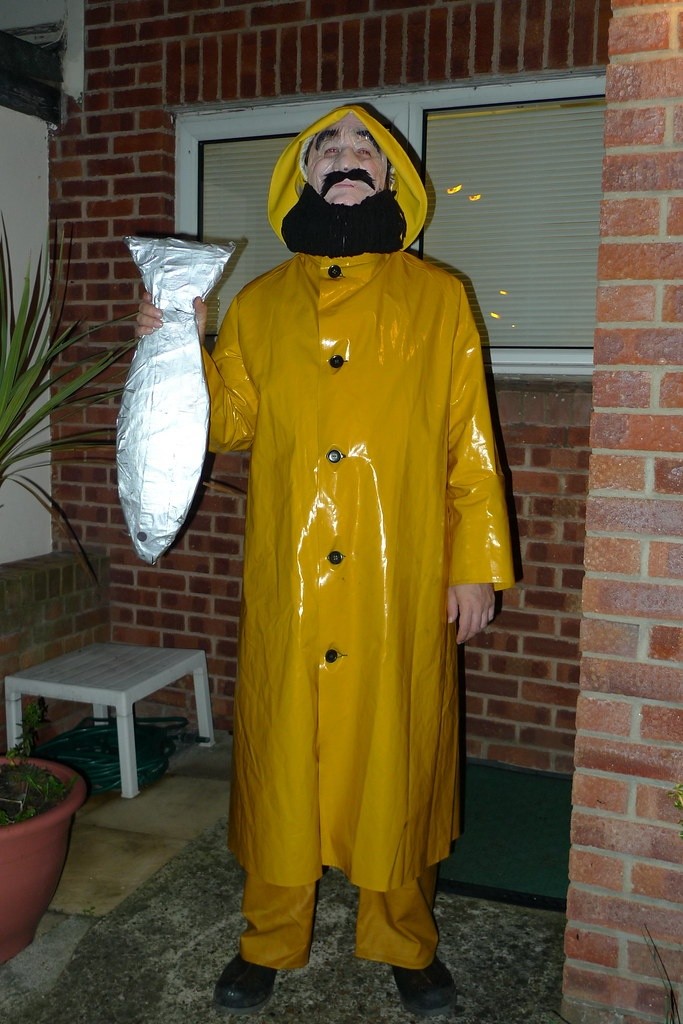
left=210, top=952, right=278, bottom=1014
left=392, top=955, right=458, bottom=1017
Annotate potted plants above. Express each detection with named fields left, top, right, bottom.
left=1, top=218, right=143, bottom=960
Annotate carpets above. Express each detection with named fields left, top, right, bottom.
left=424, top=756, right=573, bottom=912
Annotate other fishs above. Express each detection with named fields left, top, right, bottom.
left=113, top=235, right=237, bottom=563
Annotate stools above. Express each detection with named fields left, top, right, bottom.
left=4, top=642, right=216, bottom=799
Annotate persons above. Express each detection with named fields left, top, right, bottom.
left=135, top=104, right=517, bottom=1015
